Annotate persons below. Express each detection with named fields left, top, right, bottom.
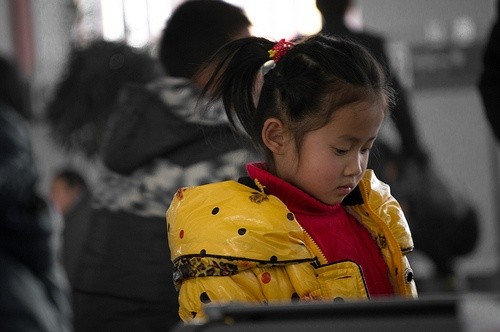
left=0, top=1, right=500, bottom=332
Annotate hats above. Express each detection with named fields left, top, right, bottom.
left=158, top=0, right=250, bottom=79
